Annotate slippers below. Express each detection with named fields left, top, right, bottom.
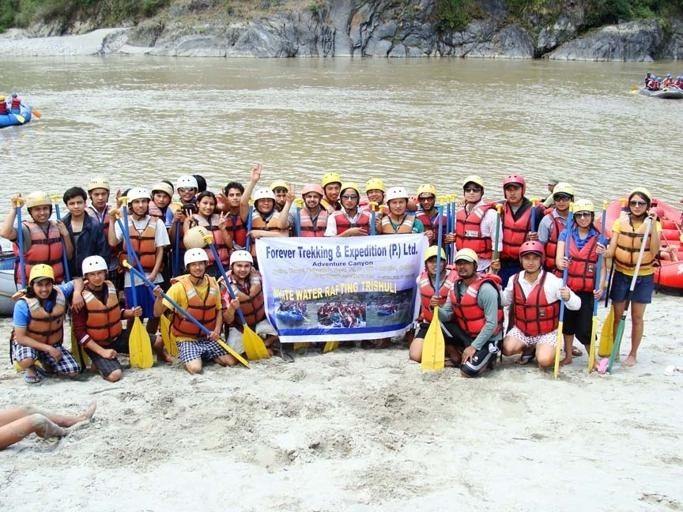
left=564, top=346, right=582, bottom=355
left=516, top=348, right=535, bottom=365
left=25, top=366, right=39, bottom=383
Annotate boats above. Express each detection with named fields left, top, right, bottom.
left=275, top=311, right=307, bottom=322
left=326, top=312, right=341, bottom=324
left=0, top=104, right=32, bottom=129
left=639, top=83, right=682, bottom=100
left=0, top=248, right=18, bottom=317
left=376, top=311, right=399, bottom=316
left=596, top=194, right=682, bottom=295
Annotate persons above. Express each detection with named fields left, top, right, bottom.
left=0, top=400, right=97, bottom=452
left=0, top=95, right=10, bottom=115
left=645, top=72, right=683, bottom=91
left=7, top=93, right=21, bottom=115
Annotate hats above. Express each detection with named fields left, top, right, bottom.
left=504, top=183, right=521, bottom=188
left=33, top=277, right=47, bottom=282
left=554, top=192, right=571, bottom=197
left=521, top=251, right=542, bottom=256
left=456, top=256, right=474, bottom=263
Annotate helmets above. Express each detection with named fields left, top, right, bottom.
left=463, top=176, right=484, bottom=191
left=82, top=255, right=108, bottom=278
left=417, top=183, right=435, bottom=198
left=504, top=174, right=525, bottom=198
left=87, top=178, right=110, bottom=191
left=553, top=182, right=574, bottom=201
left=455, top=248, right=479, bottom=264
left=26, top=191, right=51, bottom=206
left=365, top=178, right=408, bottom=203
left=573, top=199, right=594, bottom=215
left=254, top=180, right=288, bottom=202
left=519, top=241, right=544, bottom=266
left=29, top=263, right=55, bottom=286
left=302, top=172, right=360, bottom=198
left=183, top=226, right=210, bottom=248
left=628, top=187, right=652, bottom=205
left=230, top=250, right=253, bottom=266
left=184, top=248, right=209, bottom=270
left=424, top=245, right=446, bottom=261
left=126, top=174, right=198, bottom=203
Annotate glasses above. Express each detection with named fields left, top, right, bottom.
left=466, top=188, right=480, bottom=191
left=575, top=213, right=590, bottom=217
left=179, top=187, right=193, bottom=192
left=555, top=196, right=570, bottom=201
left=630, top=201, right=646, bottom=205
left=341, top=195, right=357, bottom=200
left=418, top=196, right=433, bottom=201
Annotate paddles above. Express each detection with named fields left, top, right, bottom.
left=204, top=236, right=270, bottom=362
left=121, top=258, right=250, bottom=366
left=52, top=196, right=90, bottom=367
left=323, top=201, right=390, bottom=353
left=422, top=196, right=445, bottom=370
left=116, top=195, right=177, bottom=368
left=8, top=103, right=42, bottom=123
left=293, top=199, right=311, bottom=350
left=588, top=201, right=608, bottom=372
left=606, top=204, right=656, bottom=372
left=12, top=199, right=40, bottom=373
left=554, top=203, right=573, bottom=381
left=598, top=304, right=615, bottom=356
